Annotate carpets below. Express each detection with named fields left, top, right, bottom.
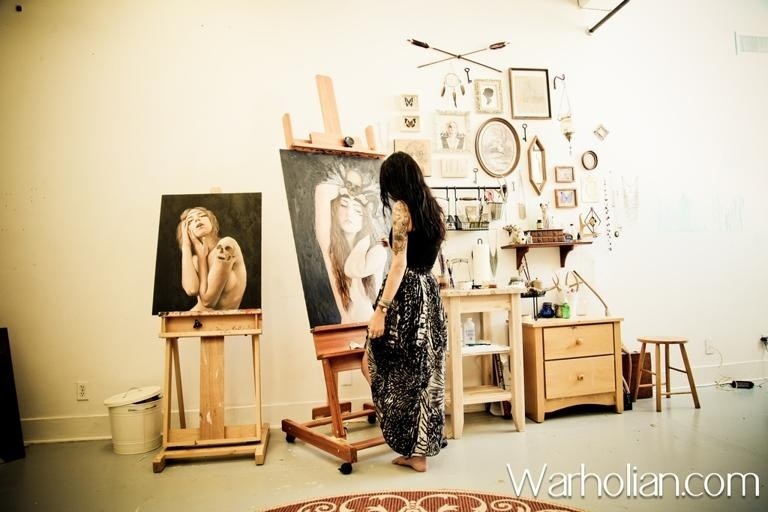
left=258, top=489, right=579, bottom=512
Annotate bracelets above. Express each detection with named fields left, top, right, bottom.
left=377, top=298, right=392, bottom=313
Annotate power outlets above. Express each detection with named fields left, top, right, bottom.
left=75, top=380, right=90, bottom=403
left=705, top=337, right=718, bottom=355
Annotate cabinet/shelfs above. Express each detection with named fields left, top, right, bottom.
left=524, top=315, right=625, bottom=424
left=438, top=285, right=527, bottom=441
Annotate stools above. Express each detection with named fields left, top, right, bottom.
left=634, top=337, right=700, bottom=413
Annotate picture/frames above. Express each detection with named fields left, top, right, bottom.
left=474, top=78, right=503, bottom=114
left=585, top=207, right=601, bottom=233
left=473, top=118, right=521, bottom=179
left=528, top=134, right=548, bottom=195
left=434, top=111, right=468, bottom=153
left=394, top=138, right=432, bottom=177
left=583, top=151, right=597, bottom=170
left=555, top=167, right=574, bottom=184
left=555, top=188, right=578, bottom=209
left=509, top=67, right=552, bottom=120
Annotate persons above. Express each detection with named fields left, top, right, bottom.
left=361, top=151, right=449, bottom=472
left=440, top=120, right=464, bottom=150
left=314, top=162, right=389, bottom=326
left=176, top=206, right=247, bottom=312
